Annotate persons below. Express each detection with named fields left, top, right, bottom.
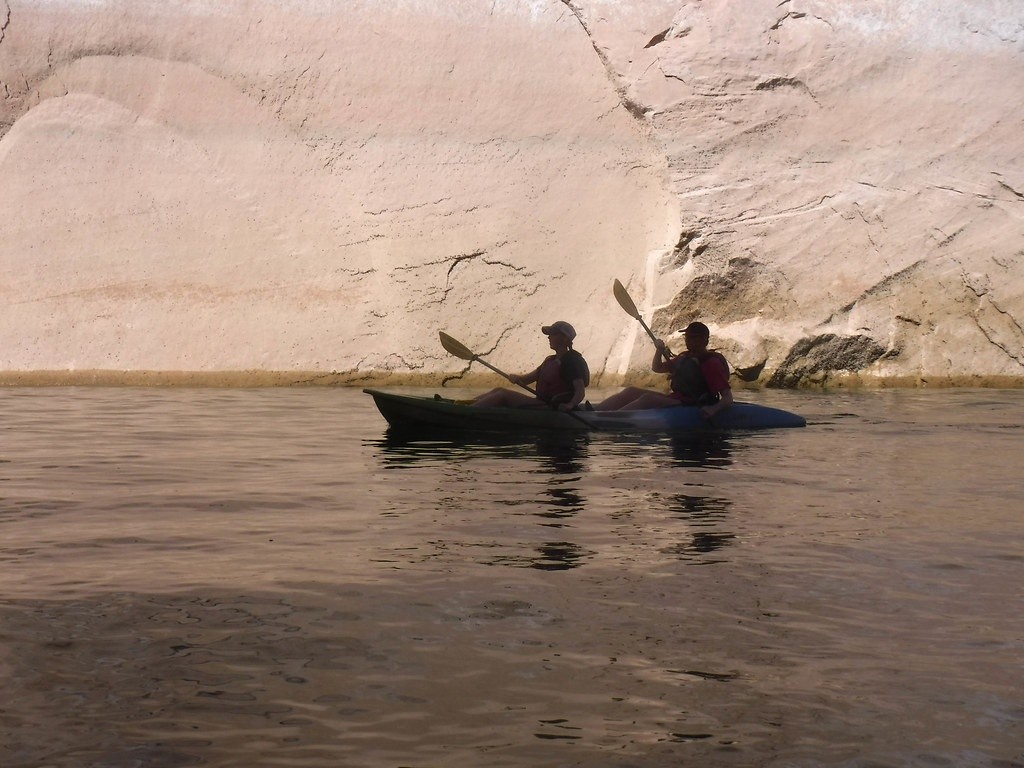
left=586, top=322, right=733, bottom=420
left=434, top=321, right=590, bottom=411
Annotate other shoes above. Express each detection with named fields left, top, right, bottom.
left=585, top=400, right=594, bottom=410
left=434, top=394, right=442, bottom=401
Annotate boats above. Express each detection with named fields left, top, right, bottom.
left=361, top=387, right=808, bottom=435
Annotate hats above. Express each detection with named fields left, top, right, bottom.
left=542, top=321, right=576, bottom=341
left=679, top=322, right=709, bottom=339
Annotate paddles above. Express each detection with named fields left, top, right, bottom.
left=439, top=330, right=601, bottom=432
left=613, top=278, right=672, bottom=363
